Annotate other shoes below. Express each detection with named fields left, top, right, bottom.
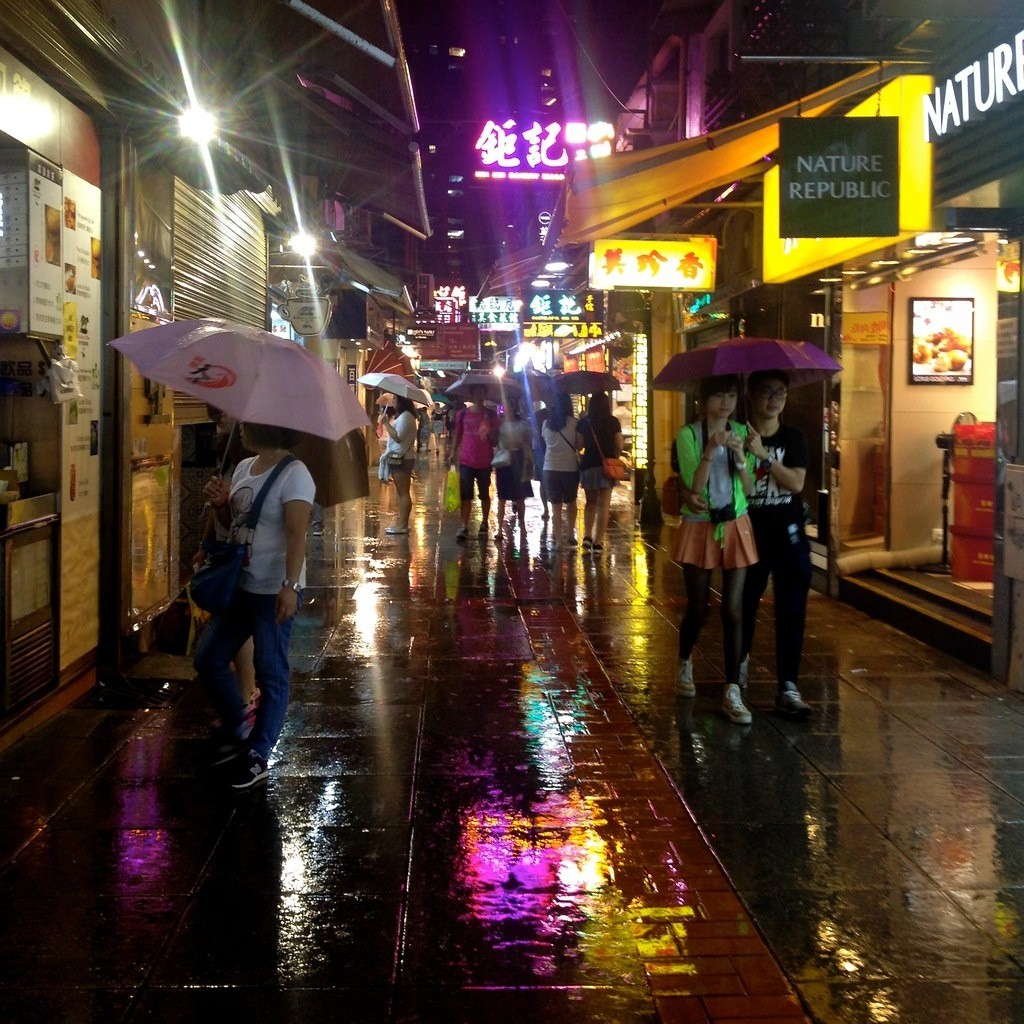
left=238, top=688, right=261, bottom=741
left=456, top=527, right=469, bottom=538
left=495, top=533, right=503, bottom=540
left=582, top=536, right=592, bottom=549
left=567, top=537, right=578, bottom=546
left=593, top=543, right=603, bottom=553
left=478, top=524, right=488, bottom=533
left=385, top=526, right=408, bottom=534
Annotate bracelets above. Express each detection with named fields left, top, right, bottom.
left=199, top=542, right=204, bottom=549
left=702, top=456, right=713, bottom=462
left=214, top=504, right=224, bottom=510
left=735, top=463, right=745, bottom=470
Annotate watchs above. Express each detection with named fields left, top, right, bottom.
left=282, top=580, right=302, bottom=593
left=764, top=454, right=774, bottom=466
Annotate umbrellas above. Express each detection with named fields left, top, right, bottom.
left=645, top=336, right=842, bottom=435
left=431, top=394, right=449, bottom=403
left=553, top=371, right=621, bottom=394
left=358, top=372, right=430, bottom=416
left=508, top=370, right=550, bottom=401
left=446, top=374, right=522, bottom=400
left=376, top=390, right=432, bottom=409
left=106, top=315, right=372, bottom=477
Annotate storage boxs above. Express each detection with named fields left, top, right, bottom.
left=949, top=525, right=993, bottom=583
left=950, top=423, right=996, bottom=475
left=948, top=475, right=996, bottom=531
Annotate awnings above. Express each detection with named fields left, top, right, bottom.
left=545, top=60, right=902, bottom=260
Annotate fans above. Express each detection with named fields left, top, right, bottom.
left=917, top=413, right=978, bottom=575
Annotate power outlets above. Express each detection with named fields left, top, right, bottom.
left=932, top=528, right=943, bottom=543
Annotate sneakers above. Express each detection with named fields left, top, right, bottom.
left=210, top=747, right=237, bottom=765
left=722, top=685, right=752, bottom=723
left=675, top=655, right=696, bottom=696
left=739, top=660, right=749, bottom=686
left=231, top=754, right=269, bottom=788
left=778, top=689, right=811, bottom=713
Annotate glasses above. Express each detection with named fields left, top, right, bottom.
left=756, top=388, right=788, bottom=400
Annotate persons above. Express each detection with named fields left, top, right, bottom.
left=431, top=403, right=446, bottom=452
left=186, top=404, right=317, bottom=788
left=441, top=394, right=622, bottom=553
left=376, top=395, right=418, bottom=535
left=672, top=370, right=813, bottom=724
left=417, top=407, right=432, bottom=452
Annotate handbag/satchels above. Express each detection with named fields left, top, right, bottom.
left=603, top=458, right=626, bottom=479
left=490, top=447, right=511, bottom=467
left=188, top=543, right=244, bottom=617
left=388, top=454, right=404, bottom=466
left=711, top=505, right=735, bottom=524
left=521, top=444, right=533, bottom=482
